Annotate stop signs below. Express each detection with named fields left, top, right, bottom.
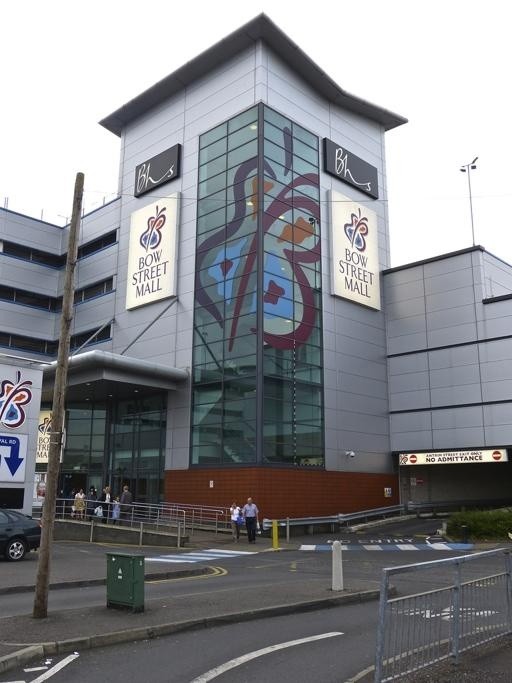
left=492, top=450, right=502, bottom=460
left=409, top=454, right=417, bottom=463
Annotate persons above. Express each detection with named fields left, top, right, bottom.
left=242, top=495, right=260, bottom=544
left=68, top=482, right=134, bottom=528
left=229, top=499, right=242, bottom=543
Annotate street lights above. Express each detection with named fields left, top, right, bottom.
left=457, top=155, right=481, bottom=249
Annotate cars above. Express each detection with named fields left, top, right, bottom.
left=0, top=507, right=41, bottom=560
